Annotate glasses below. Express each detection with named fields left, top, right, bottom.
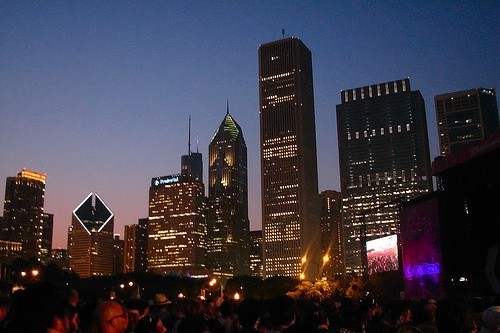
left=106, top=307, right=128, bottom=324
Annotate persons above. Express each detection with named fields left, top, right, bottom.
left=0, top=277, right=500, bottom=333
